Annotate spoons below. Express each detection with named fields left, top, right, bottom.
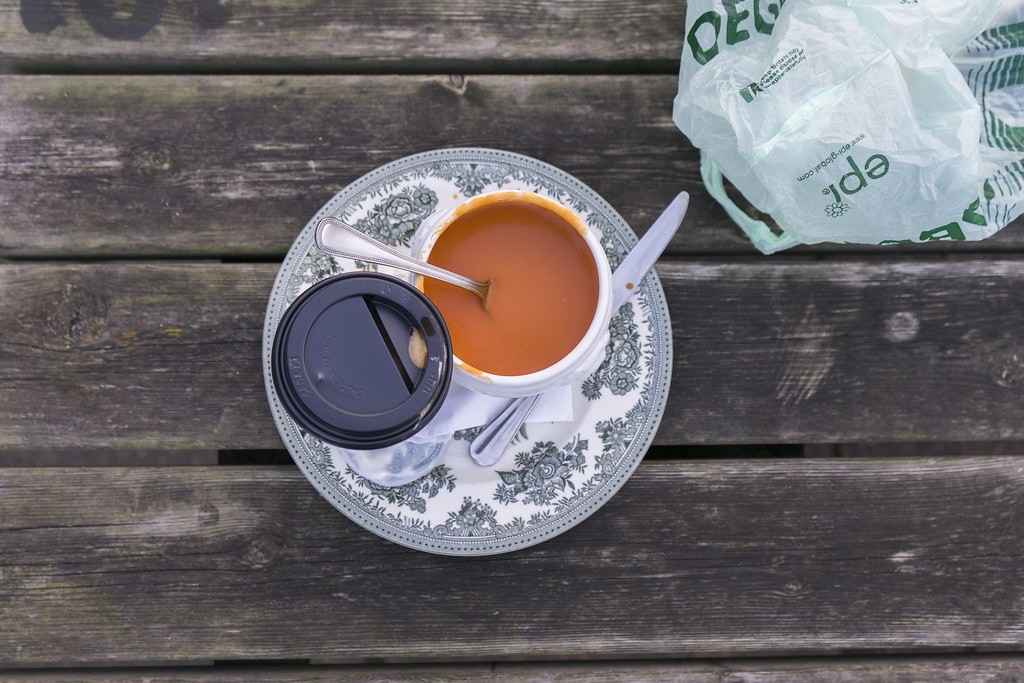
left=314, top=214, right=493, bottom=312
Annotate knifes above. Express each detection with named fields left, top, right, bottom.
left=468, top=191, right=690, bottom=466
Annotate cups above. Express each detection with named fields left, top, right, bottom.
left=271, top=272, right=454, bottom=486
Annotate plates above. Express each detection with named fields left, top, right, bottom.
left=261, top=147, right=674, bottom=556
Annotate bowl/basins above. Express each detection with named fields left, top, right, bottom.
left=403, top=190, right=613, bottom=398
